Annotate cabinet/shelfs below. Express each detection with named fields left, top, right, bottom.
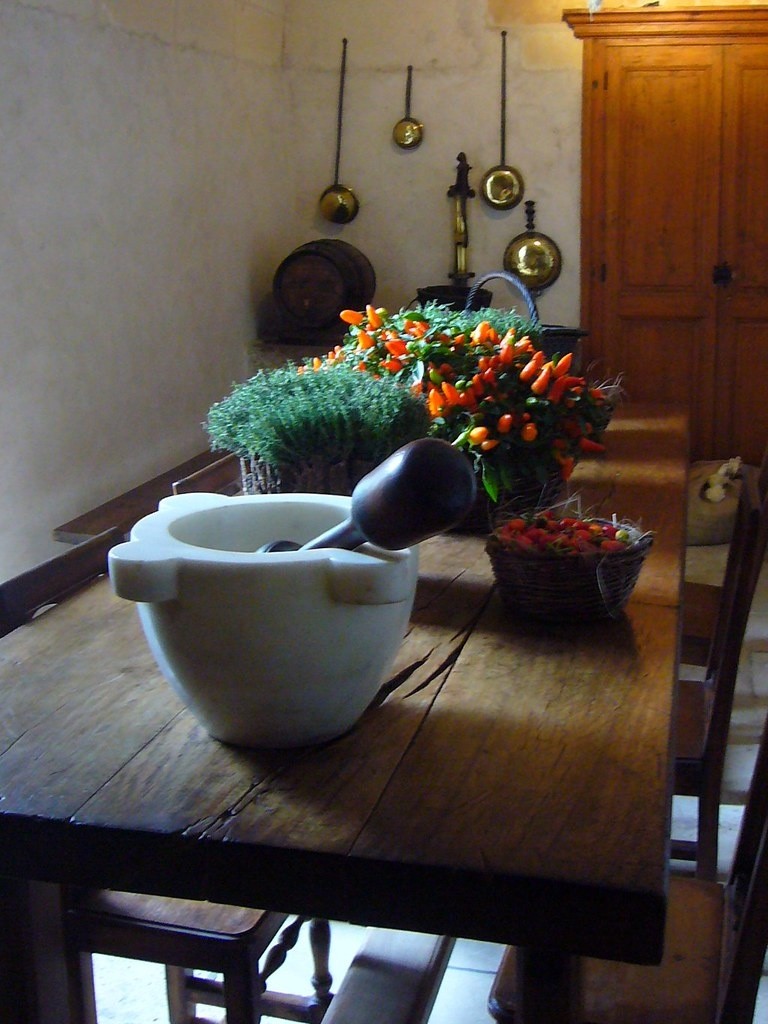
left=561, top=8, right=768, bottom=466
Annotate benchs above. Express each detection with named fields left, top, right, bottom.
left=50, top=443, right=240, bottom=541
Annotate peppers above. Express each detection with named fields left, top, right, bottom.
left=297, top=307, right=606, bottom=481
left=500, top=511, right=628, bottom=555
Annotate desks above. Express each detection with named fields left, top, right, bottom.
left=0, top=400, right=693, bottom=1024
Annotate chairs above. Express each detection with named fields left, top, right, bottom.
left=486, top=450, right=767, bottom=1024
left=0, top=446, right=336, bottom=1024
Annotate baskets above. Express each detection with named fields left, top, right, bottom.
left=461, top=271, right=589, bottom=375
left=453, top=467, right=574, bottom=536
left=579, top=384, right=622, bottom=459
left=485, top=513, right=655, bottom=624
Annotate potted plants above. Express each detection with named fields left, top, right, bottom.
left=200, top=354, right=431, bottom=494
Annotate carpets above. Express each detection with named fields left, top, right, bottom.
left=686, top=459, right=758, bottom=546
